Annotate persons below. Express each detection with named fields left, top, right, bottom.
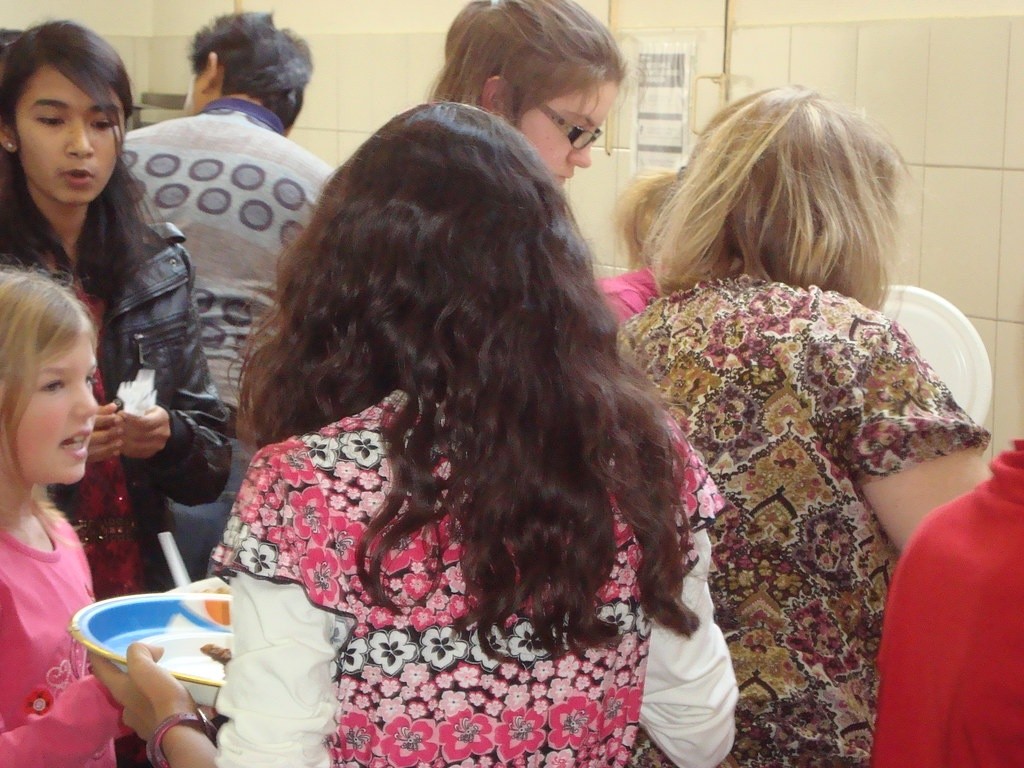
left=120, top=14, right=335, bottom=596
left=425, top=0, right=627, bottom=189
left=869, top=439, right=1024, bottom=768
left=0, top=20, right=231, bottom=768
left=0, top=266, right=137, bottom=768
left=588, top=168, right=685, bottom=330
left=90, top=100, right=738, bottom=768
left=616, top=84, right=994, bottom=768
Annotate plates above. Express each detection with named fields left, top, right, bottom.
left=66, top=591, right=233, bottom=708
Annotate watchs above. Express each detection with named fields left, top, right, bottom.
left=145, top=707, right=218, bottom=768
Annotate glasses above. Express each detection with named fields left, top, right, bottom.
left=536, top=105, right=603, bottom=148
